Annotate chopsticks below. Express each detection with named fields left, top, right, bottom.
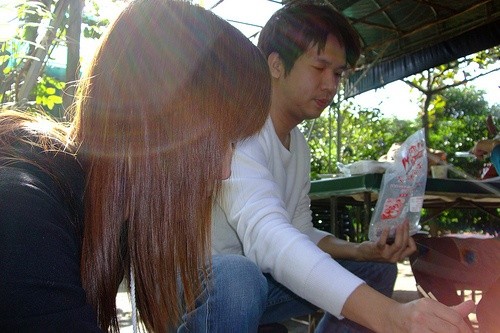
left=417, top=284, right=439, bottom=303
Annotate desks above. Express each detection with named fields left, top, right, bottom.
left=307, top=173, right=500, bottom=243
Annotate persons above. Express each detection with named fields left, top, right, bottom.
left=0, top=0, right=271, bottom=332
left=127, top=0, right=475, bottom=333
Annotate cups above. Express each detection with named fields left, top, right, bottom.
left=431, top=166, right=447, bottom=178
